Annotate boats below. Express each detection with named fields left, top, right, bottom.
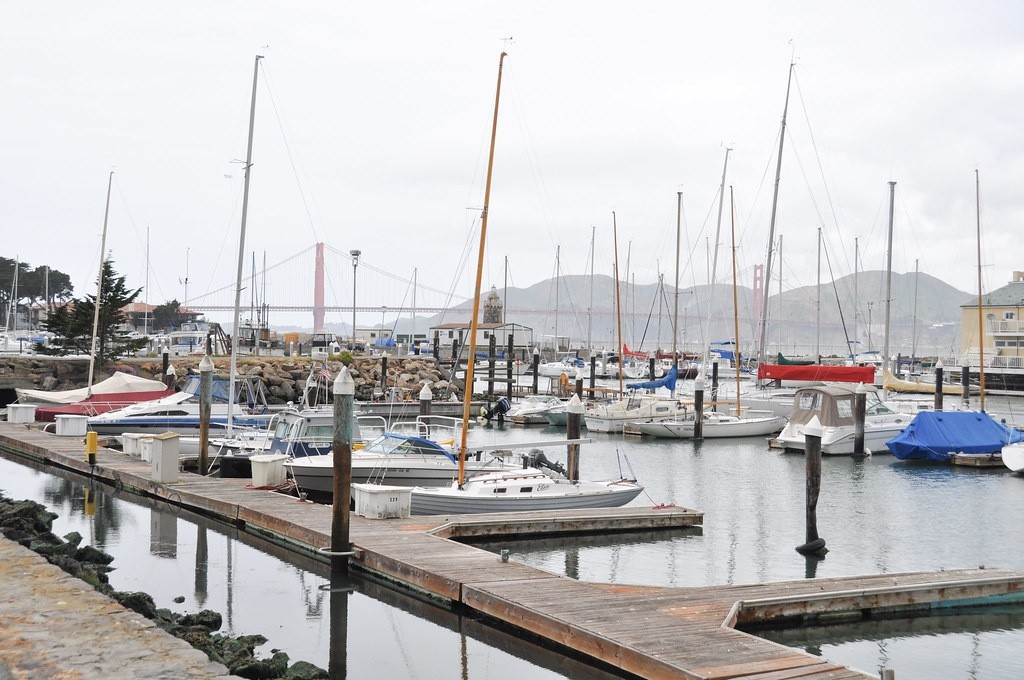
left=283, top=419, right=567, bottom=506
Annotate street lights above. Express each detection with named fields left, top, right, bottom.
left=349, top=249, right=361, bottom=351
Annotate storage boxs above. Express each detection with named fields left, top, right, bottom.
left=6, top=404, right=38, bottom=424
left=122, top=433, right=154, bottom=463
left=55, top=415, right=88, bottom=436
left=249, top=454, right=290, bottom=488
left=353, top=484, right=414, bottom=519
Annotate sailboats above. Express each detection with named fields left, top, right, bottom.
left=8, top=48, right=1024, bottom=471
left=350, top=50, right=645, bottom=517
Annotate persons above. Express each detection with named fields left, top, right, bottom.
left=206, top=333, right=212, bottom=355
left=226, top=334, right=232, bottom=354
